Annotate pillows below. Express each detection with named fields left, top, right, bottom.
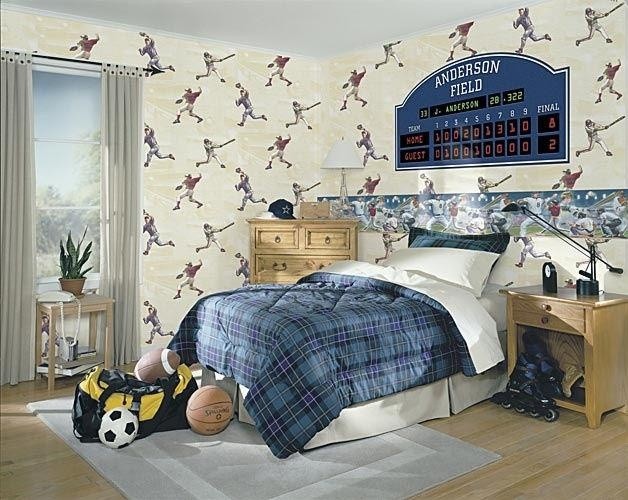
left=381, top=247, right=500, bottom=299
left=408, top=227, right=511, bottom=276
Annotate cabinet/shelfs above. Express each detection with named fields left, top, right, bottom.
left=247, top=220, right=358, bottom=284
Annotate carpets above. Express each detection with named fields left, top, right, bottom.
left=26, top=370, right=502, bottom=500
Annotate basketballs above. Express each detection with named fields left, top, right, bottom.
left=186, top=386, right=233, bottom=435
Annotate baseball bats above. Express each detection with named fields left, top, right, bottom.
left=305, top=181, right=321, bottom=191
left=498, top=174, right=512, bottom=184
left=307, top=102, right=322, bottom=110
left=220, top=53, right=235, bottom=62
left=588, top=192, right=621, bottom=212
left=221, top=222, right=235, bottom=231
left=607, top=115, right=625, bottom=130
left=605, top=2, right=624, bottom=19
left=219, top=139, right=235, bottom=147
left=391, top=195, right=416, bottom=213
left=400, top=234, right=408, bottom=240
left=479, top=194, right=502, bottom=210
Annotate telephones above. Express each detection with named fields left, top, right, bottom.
left=35, top=290, right=82, bottom=346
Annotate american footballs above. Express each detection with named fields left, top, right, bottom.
left=132, top=348, right=180, bottom=384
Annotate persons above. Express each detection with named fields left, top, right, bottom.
left=142, top=209, right=174, bottom=254
left=172, top=172, right=202, bottom=211
left=355, top=127, right=389, bottom=167
left=572, top=210, right=576, bottom=224
left=234, top=256, right=251, bottom=287
left=375, top=39, right=403, bottom=69
left=264, top=134, right=292, bottom=170
left=137, top=32, right=175, bottom=77
left=173, top=259, right=204, bottom=300
left=594, top=58, right=622, bottom=103
left=487, top=209, right=506, bottom=233
left=143, top=304, right=174, bottom=344
left=596, top=208, right=621, bottom=238
left=265, top=55, right=292, bottom=87
left=512, top=7, right=551, bottom=53
left=400, top=209, right=415, bottom=233
left=195, top=51, right=225, bottom=83
left=143, top=123, right=175, bottom=167
left=419, top=177, right=435, bottom=195
left=382, top=213, right=398, bottom=233
left=290, top=183, right=307, bottom=207
left=422, top=194, right=455, bottom=231
left=574, top=8, right=612, bottom=46
left=468, top=212, right=485, bottom=235
left=195, top=223, right=225, bottom=253
left=339, top=66, right=367, bottom=111
left=557, top=192, right=575, bottom=214
left=234, top=85, right=267, bottom=126
left=195, top=138, right=225, bottom=169
left=613, top=190, right=627, bottom=217
left=446, top=21, right=476, bottom=63
left=441, top=202, right=466, bottom=232
left=172, top=86, right=203, bottom=124
left=456, top=195, right=477, bottom=231
left=569, top=213, right=596, bottom=237
left=477, top=177, right=496, bottom=203
left=499, top=194, right=509, bottom=217
left=519, top=192, right=557, bottom=236
left=234, top=170, right=267, bottom=211
left=535, top=200, right=569, bottom=235
left=575, top=120, right=612, bottom=156
left=362, top=203, right=381, bottom=230
left=349, top=197, right=368, bottom=231
left=551, top=166, right=583, bottom=190
left=69, top=33, right=99, bottom=59
left=358, top=173, right=381, bottom=195
left=513, top=237, right=551, bottom=268
left=285, top=101, right=311, bottom=130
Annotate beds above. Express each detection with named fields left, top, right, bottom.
left=168, top=260, right=507, bottom=460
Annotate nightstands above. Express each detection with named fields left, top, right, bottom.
left=499, top=284, right=628, bottom=428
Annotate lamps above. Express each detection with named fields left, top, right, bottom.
left=322, top=137, right=364, bottom=218
left=502, top=202, right=623, bottom=298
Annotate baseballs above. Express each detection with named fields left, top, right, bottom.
left=617, top=57, right=620, bottom=61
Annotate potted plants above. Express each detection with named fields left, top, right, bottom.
left=58, top=227, right=94, bottom=296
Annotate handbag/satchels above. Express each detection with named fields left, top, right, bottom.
left=71, top=363, right=199, bottom=444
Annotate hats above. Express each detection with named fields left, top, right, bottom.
left=268, top=199, right=296, bottom=219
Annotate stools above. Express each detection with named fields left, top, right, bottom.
left=35, top=295, right=115, bottom=390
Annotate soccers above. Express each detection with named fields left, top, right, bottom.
left=97, top=408, right=140, bottom=449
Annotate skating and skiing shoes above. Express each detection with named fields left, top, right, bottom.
left=491, top=352, right=559, bottom=423
left=522, top=331, right=565, bottom=398
